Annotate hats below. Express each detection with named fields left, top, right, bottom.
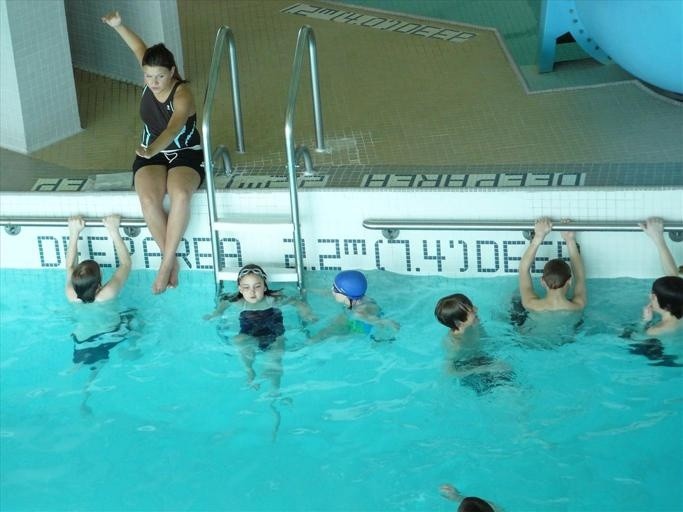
left=334, top=270, right=367, bottom=298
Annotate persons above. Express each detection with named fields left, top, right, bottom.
left=438, top=481, right=505, bottom=511
left=579, top=215, right=683, bottom=367
left=432, top=293, right=519, bottom=393
left=198, top=263, right=319, bottom=443
left=98, top=9, right=207, bottom=295
left=303, top=268, right=401, bottom=360
left=62, top=212, right=147, bottom=418
left=493, top=215, right=590, bottom=352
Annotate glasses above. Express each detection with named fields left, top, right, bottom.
left=334, top=287, right=346, bottom=296
left=238, top=269, right=262, bottom=276
